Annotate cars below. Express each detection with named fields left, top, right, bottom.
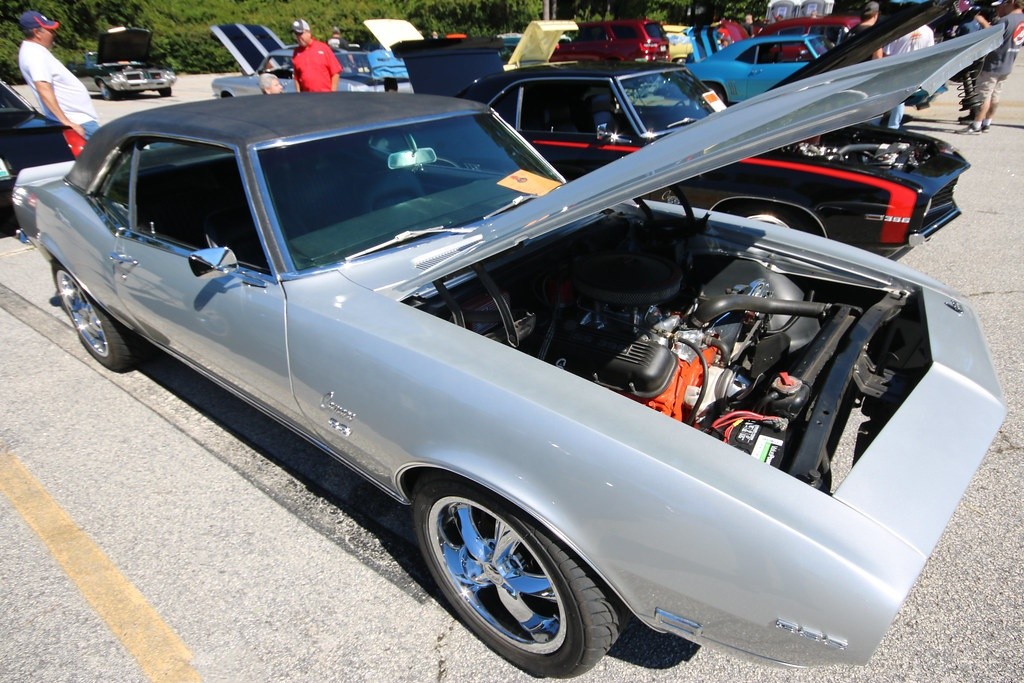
left=64, top=25, right=176, bottom=101
left=210, top=0, right=972, bottom=251
left=0, top=80, right=89, bottom=209
left=11, top=23, right=1005, bottom=678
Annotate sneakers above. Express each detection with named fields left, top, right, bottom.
left=954, top=123, right=989, bottom=135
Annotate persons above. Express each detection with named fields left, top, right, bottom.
left=326, top=25, right=355, bottom=72
left=869, top=2, right=936, bottom=131
left=946, top=0, right=1024, bottom=135
left=18, top=12, right=100, bottom=141
left=742, top=15, right=754, bottom=40
left=843, top=2, right=882, bottom=61
left=258, top=73, right=284, bottom=95
left=288, top=19, right=343, bottom=93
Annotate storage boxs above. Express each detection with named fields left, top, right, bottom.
left=391, top=36, right=504, bottom=97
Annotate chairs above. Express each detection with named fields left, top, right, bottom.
left=109, top=139, right=389, bottom=260
left=527, top=91, right=614, bottom=132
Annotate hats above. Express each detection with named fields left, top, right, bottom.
left=991, top=0, right=1005, bottom=7
left=861, top=1, right=880, bottom=14
left=18, top=11, right=59, bottom=30
left=289, top=18, right=309, bottom=33
